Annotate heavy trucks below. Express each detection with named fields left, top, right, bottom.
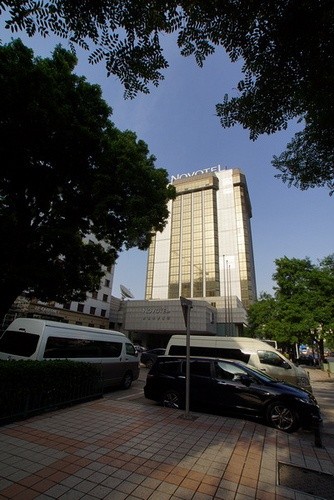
left=298, top=345, right=308, bottom=355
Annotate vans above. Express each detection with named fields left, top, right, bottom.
left=144, top=357, right=323, bottom=433
left=164, top=334, right=312, bottom=406
left=0, top=318, right=169, bottom=409
